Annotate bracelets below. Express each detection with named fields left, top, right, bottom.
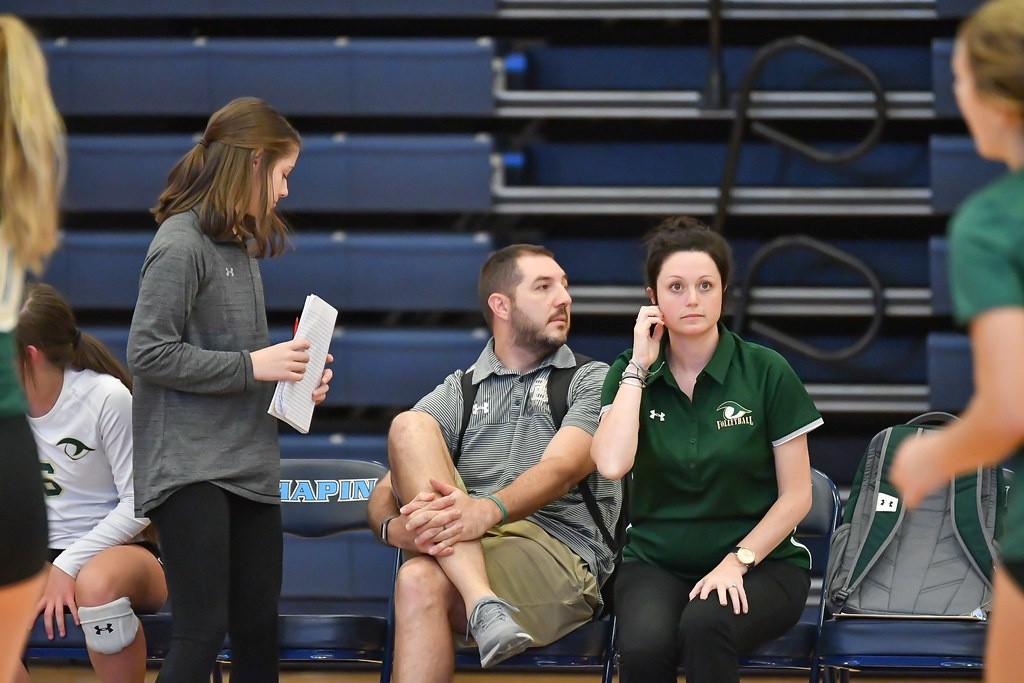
left=485, top=495, right=507, bottom=528
left=618, top=359, right=665, bottom=388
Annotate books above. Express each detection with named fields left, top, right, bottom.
left=268, top=293, right=338, bottom=434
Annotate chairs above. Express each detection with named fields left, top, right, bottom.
left=22, top=456, right=1004, bottom=683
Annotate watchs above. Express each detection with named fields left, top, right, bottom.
left=730, top=546, right=756, bottom=572
left=379, top=515, right=398, bottom=546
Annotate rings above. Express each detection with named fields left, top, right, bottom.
left=443, top=523, right=447, bottom=530
left=728, top=584, right=736, bottom=589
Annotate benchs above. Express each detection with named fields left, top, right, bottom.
left=927, top=0, right=1009, bottom=427
left=28, top=0, right=499, bottom=460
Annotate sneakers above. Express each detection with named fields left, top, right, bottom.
left=466, top=596, right=533, bottom=668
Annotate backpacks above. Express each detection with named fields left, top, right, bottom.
left=824, top=411, right=1008, bottom=623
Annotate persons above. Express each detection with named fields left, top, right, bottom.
left=125, top=94, right=335, bottom=683
left=590, top=218, right=827, bottom=683
left=888, top=0, right=1024, bottom=683
left=367, top=244, right=623, bottom=683
left=0, top=11, right=170, bottom=683
